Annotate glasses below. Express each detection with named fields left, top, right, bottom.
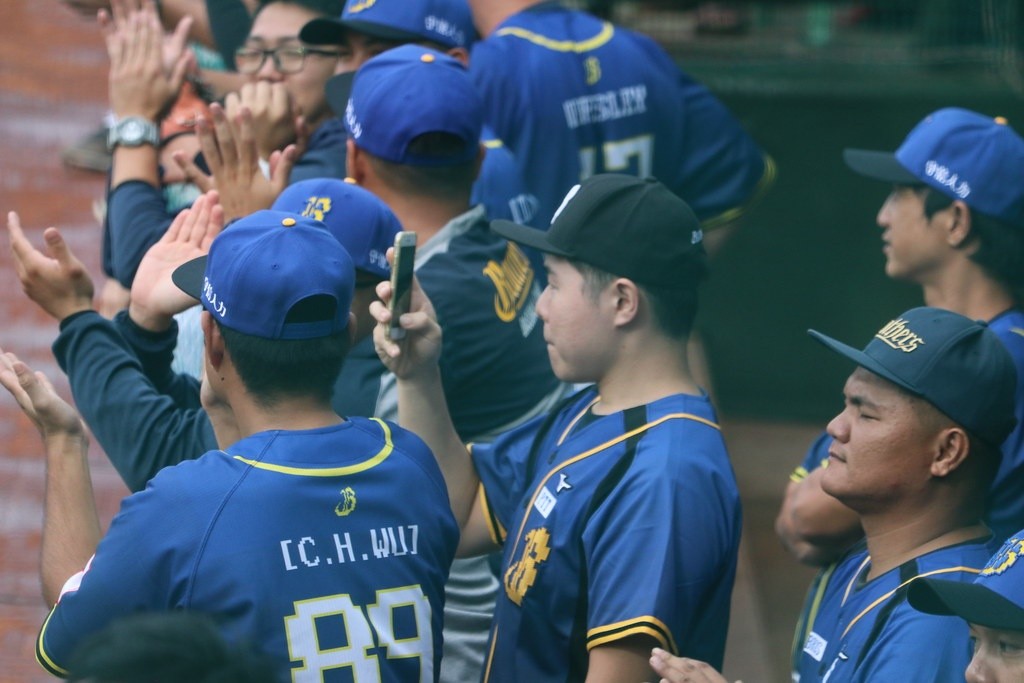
left=234, top=48, right=340, bottom=74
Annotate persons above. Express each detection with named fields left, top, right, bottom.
left=0, top=0, right=744, bottom=683
left=648, top=105, right=1024, bottom=683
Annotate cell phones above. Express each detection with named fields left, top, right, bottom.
left=385, top=230, right=419, bottom=340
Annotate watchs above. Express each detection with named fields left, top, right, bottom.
left=105, top=116, right=160, bottom=153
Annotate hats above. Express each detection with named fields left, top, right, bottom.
left=271, top=175, right=403, bottom=280
left=299, top=0, right=478, bottom=50
left=171, top=210, right=356, bottom=335
left=907, top=529, right=1024, bottom=631
left=490, top=172, right=704, bottom=296
left=843, top=107, right=1023, bottom=218
left=342, top=43, right=485, bottom=163
left=809, top=307, right=1014, bottom=484
